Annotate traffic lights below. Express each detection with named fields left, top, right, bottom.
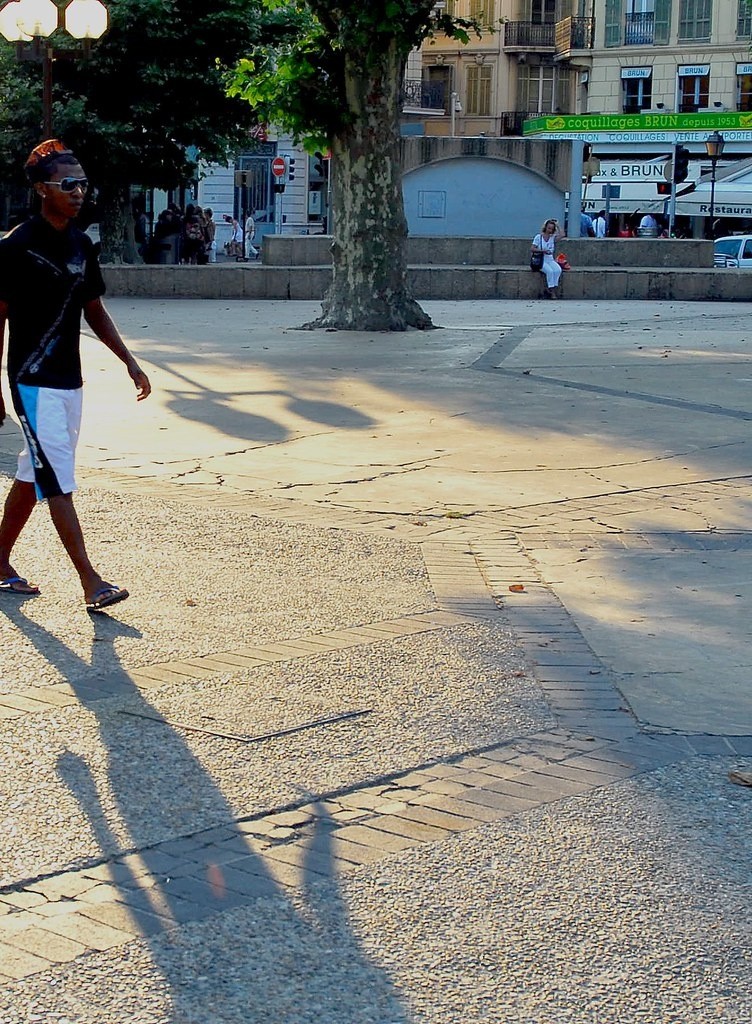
left=656, top=182, right=671, bottom=194
left=675, top=144, right=689, bottom=184
left=313, top=151, right=331, bottom=178
left=285, top=154, right=295, bottom=184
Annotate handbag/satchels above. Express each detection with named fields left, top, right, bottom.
left=185, top=222, right=205, bottom=241
left=529, top=234, right=543, bottom=269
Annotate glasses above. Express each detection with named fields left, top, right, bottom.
left=42, top=177, right=88, bottom=195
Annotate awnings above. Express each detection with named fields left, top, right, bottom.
left=565, top=182, right=752, bottom=218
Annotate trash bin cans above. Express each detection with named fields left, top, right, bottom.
left=158, top=233, right=178, bottom=264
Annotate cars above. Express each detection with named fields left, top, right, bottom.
left=713, top=234, right=752, bottom=268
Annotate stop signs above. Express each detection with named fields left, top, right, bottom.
left=273, top=157, right=286, bottom=176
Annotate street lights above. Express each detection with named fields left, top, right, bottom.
left=705, top=127, right=727, bottom=241
left=0, top=0, right=107, bottom=148
left=450, top=90, right=463, bottom=137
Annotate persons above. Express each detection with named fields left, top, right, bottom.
left=226, top=210, right=259, bottom=262
left=531, top=219, right=565, bottom=300
left=581, top=206, right=609, bottom=237
left=134, top=202, right=215, bottom=264
left=0, top=140, right=151, bottom=610
left=712, top=218, right=721, bottom=238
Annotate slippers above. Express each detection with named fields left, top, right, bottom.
left=0, top=577, right=40, bottom=595
left=85, top=586, right=129, bottom=610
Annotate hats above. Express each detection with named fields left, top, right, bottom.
left=23, top=138, right=73, bottom=180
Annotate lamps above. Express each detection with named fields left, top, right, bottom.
left=713, top=101, right=723, bottom=107
left=657, top=102, right=664, bottom=110
